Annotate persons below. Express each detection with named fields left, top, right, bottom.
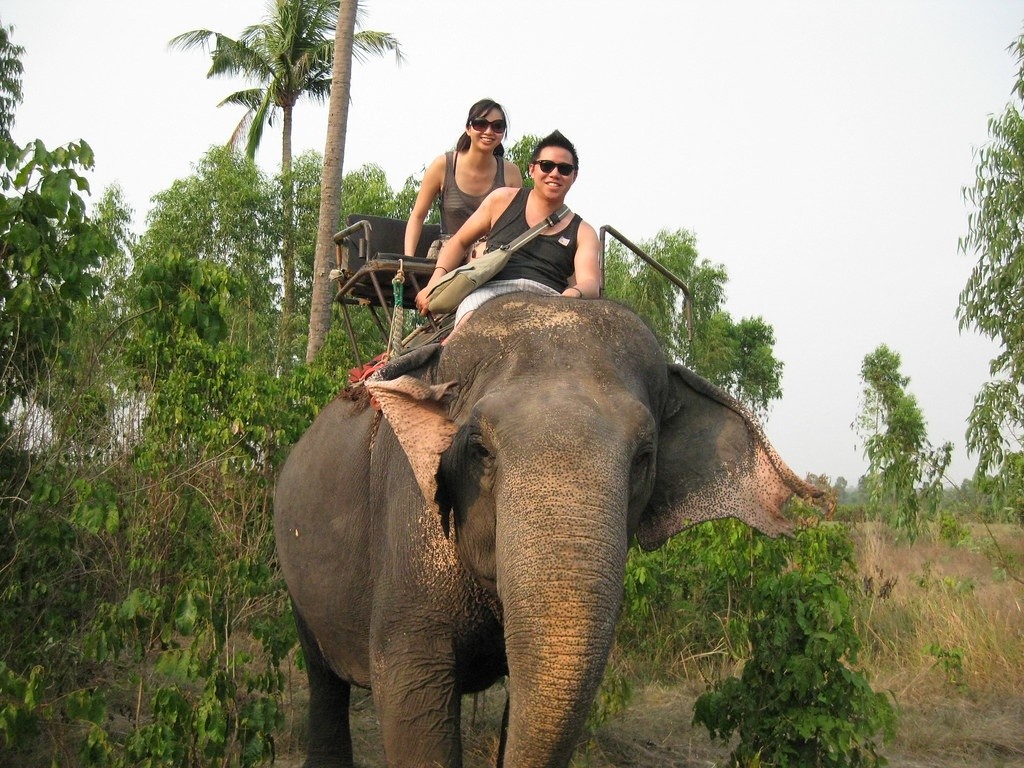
left=415, top=129, right=598, bottom=345
left=405, top=99, right=523, bottom=259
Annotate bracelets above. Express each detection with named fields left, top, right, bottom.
left=435, top=266, right=447, bottom=273
left=573, top=287, right=582, bottom=297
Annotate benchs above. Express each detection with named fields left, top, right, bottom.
left=333, top=214, right=441, bottom=373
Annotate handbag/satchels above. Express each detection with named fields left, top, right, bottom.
left=422, top=202, right=572, bottom=313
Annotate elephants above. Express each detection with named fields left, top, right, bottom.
left=273, top=290, right=826, bottom=768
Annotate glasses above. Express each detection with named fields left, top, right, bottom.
left=469, top=117, right=507, bottom=133
left=533, top=160, right=576, bottom=176
